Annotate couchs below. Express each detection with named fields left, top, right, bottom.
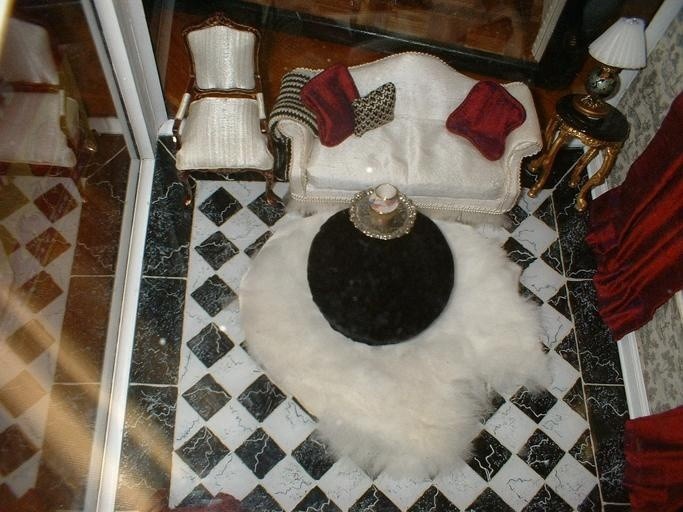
left=268, top=50, right=543, bottom=216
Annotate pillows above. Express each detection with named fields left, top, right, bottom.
left=301, top=61, right=525, bottom=161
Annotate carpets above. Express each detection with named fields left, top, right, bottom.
left=233, top=208, right=547, bottom=484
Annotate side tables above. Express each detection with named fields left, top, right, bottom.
left=526, top=93, right=630, bottom=212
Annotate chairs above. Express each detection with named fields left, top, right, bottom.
left=0, top=21, right=98, bottom=206
left=167, top=12, right=278, bottom=208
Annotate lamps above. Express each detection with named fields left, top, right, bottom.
left=570, top=16, right=647, bottom=123
